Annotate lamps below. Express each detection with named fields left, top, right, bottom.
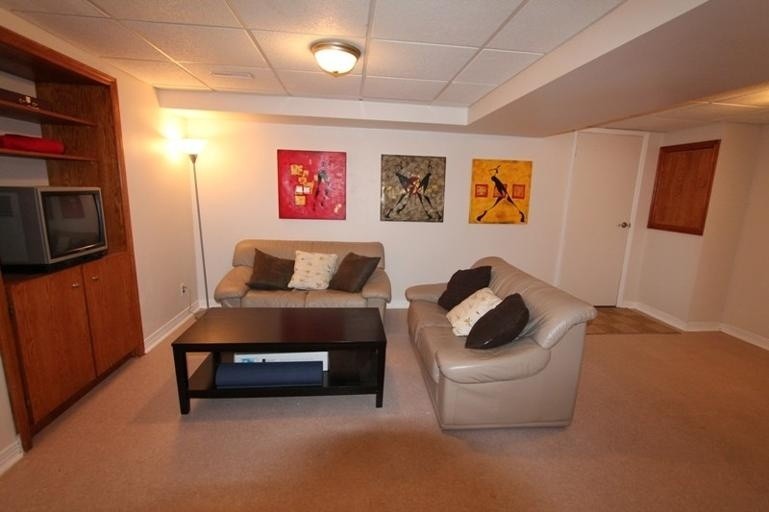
left=309, top=40, right=361, bottom=76
left=181, top=140, right=210, bottom=308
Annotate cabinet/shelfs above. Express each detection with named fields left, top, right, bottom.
left=0, top=25, right=129, bottom=287
left=0, top=249, right=147, bottom=452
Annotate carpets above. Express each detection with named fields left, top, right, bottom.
left=584, top=307, right=681, bottom=335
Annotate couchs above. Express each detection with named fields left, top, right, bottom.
left=214, top=240, right=391, bottom=327
left=406, top=257, right=597, bottom=432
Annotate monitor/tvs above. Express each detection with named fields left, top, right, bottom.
left=0, top=184, right=108, bottom=265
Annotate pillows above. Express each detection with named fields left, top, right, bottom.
left=445, top=287, right=502, bottom=337
left=287, top=250, right=337, bottom=290
left=245, top=247, right=294, bottom=290
left=438, top=266, right=492, bottom=310
left=328, top=251, right=381, bottom=292
left=465, top=293, right=529, bottom=349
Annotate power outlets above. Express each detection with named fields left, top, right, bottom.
left=180, top=284, right=187, bottom=295
